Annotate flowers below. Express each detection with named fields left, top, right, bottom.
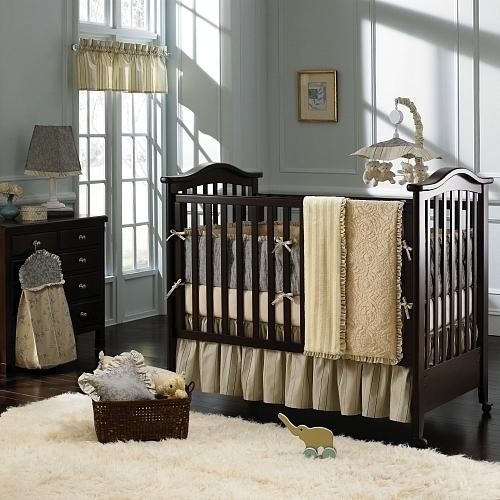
left=0, top=182, right=24, bottom=201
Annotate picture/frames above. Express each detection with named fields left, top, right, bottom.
left=296, top=69, right=339, bottom=123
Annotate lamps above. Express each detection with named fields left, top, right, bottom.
left=23, top=124, right=84, bottom=221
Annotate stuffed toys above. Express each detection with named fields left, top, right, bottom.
left=348, top=102, right=445, bottom=186
left=157, top=375, right=188, bottom=400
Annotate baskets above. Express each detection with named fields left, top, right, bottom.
left=92, top=382, right=195, bottom=444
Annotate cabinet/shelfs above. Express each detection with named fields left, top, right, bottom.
left=1, top=214, right=109, bottom=380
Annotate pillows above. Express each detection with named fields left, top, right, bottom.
left=77, top=350, right=178, bottom=402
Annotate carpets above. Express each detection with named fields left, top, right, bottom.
left=0, top=389, right=500, bottom=500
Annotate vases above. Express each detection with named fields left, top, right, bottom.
left=0, top=192, right=21, bottom=221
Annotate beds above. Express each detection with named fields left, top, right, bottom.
left=159, top=161, right=495, bottom=449
